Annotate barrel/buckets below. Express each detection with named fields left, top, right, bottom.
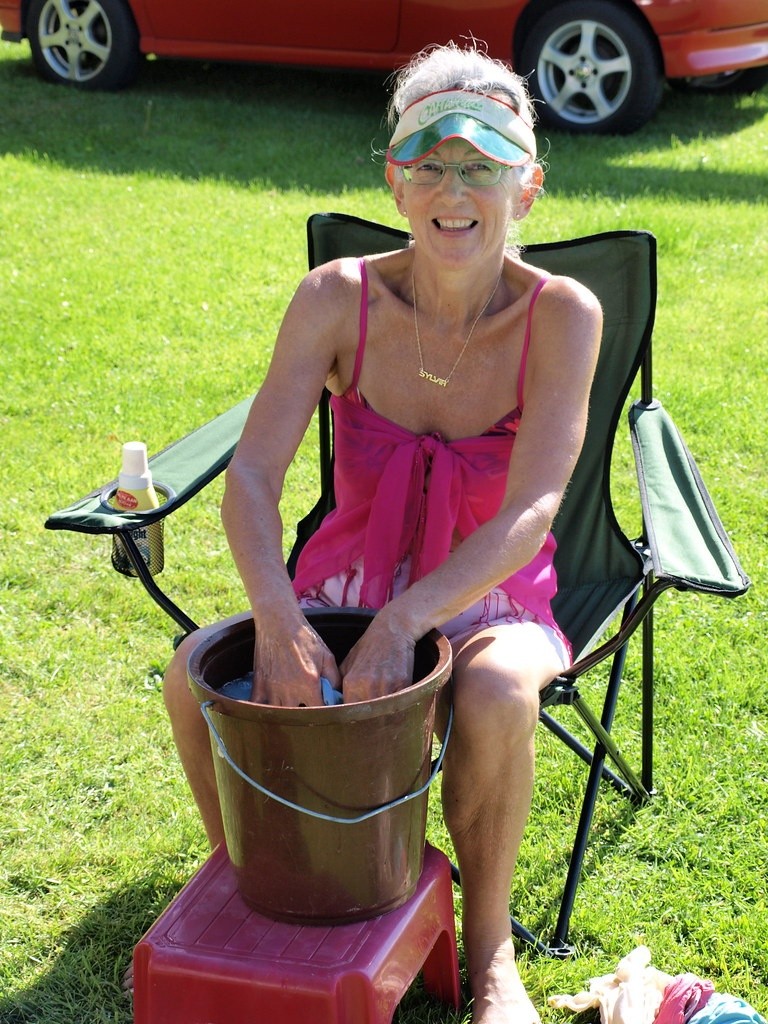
left=185, top=606, right=457, bottom=928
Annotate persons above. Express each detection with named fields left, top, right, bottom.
left=221, top=42, right=604, bottom=1024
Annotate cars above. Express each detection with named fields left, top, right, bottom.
left=4, top=1, right=766, bottom=136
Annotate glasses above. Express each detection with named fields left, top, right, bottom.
left=400, top=158, right=511, bottom=185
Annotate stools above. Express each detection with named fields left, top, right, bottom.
left=132, top=837, right=463, bottom=1024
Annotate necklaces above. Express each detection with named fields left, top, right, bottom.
left=412, top=259, right=505, bottom=386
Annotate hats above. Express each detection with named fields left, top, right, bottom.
left=386, top=89, right=537, bottom=168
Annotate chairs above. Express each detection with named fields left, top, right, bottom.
left=43, top=209, right=755, bottom=965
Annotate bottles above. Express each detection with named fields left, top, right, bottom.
left=109, top=440, right=165, bottom=579
